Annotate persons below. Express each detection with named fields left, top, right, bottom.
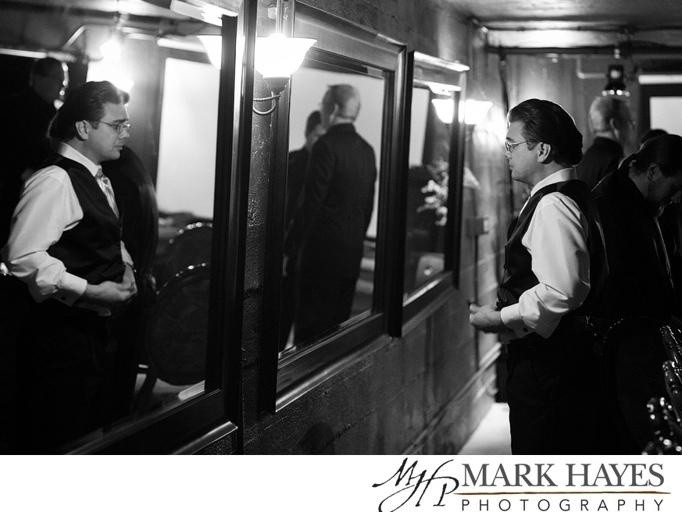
left=572, top=94, right=682, bottom=455
left=279, top=85, right=377, bottom=348
left=468, top=98, right=618, bottom=454
left=279, top=110, right=329, bottom=356
left=1, top=57, right=158, bottom=454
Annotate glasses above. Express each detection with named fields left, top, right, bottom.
left=98, top=121, right=131, bottom=133
left=504, top=142, right=525, bottom=151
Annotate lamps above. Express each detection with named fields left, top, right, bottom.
left=198, top=35, right=243, bottom=99
left=432, top=98, right=454, bottom=128
left=246, top=38, right=316, bottom=115
left=467, top=98, right=492, bottom=133
